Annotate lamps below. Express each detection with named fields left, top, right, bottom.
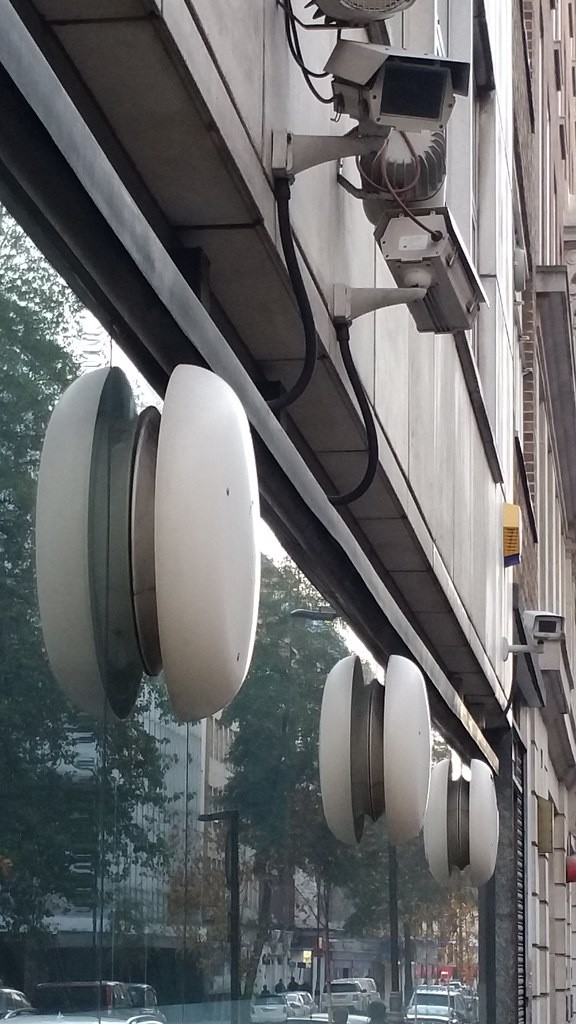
left=365, top=651, right=433, bottom=837
left=316, top=652, right=368, bottom=847
left=126, top=358, right=260, bottom=721
left=459, top=757, right=498, bottom=887
left=35, top=366, right=142, bottom=724
left=423, top=758, right=457, bottom=884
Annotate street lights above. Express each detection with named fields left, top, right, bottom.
left=197, top=810, right=240, bottom=1024
left=292, top=607, right=401, bottom=994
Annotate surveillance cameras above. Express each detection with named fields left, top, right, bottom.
left=370, top=204, right=491, bottom=335
left=322, top=40, right=472, bottom=131
left=524, top=610, right=565, bottom=639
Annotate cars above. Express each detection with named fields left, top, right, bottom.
left=250, top=993, right=295, bottom=1024
left=0, top=980, right=167, bottom=1022
left=321, top=977, right=382, bottom=1013
left=403, top=980, right=478, bottom=1024
left=270, top=995, right=310, bottom=1020
left=297, top=991, right=318, bottom=1014
